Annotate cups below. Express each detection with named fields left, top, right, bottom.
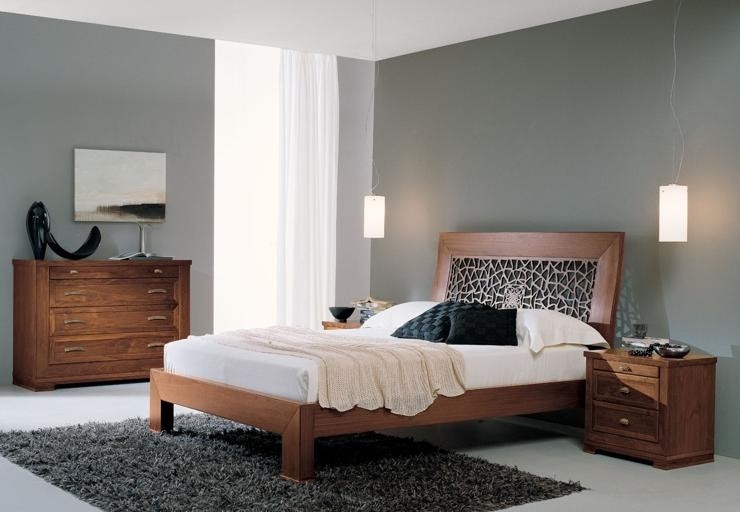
left=632, top=323, right=648, bottom=340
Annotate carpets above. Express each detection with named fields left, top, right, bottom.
left=0, top=411, right=593, bottom=511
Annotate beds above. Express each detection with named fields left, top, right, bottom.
left=149, top=231, right=625, bottom=486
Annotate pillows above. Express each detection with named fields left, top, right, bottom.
left=358, top=300, right=612, bottom=354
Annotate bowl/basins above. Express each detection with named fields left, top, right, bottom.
left=329, top=306, right=355, bottom=322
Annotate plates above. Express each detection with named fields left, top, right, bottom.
left=656, top=343, right=690, bottom=358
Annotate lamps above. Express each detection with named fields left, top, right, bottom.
left=657, top=0, right=689, bottom=244
left=362, top=1, right=387, bottom=240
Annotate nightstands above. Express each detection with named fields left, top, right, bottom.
left=320, top=321, right=361, bottom=331
left=582, top=348, right=718, bottom=470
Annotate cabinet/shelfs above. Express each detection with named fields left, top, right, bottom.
left=11, top=258, right=193, bottom=394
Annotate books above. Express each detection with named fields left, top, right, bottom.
left=108, top=252, right=173, bottom=261
left=352, top=296, right=394, bottom=324
left=129, top=256, right=173, bottom=260
